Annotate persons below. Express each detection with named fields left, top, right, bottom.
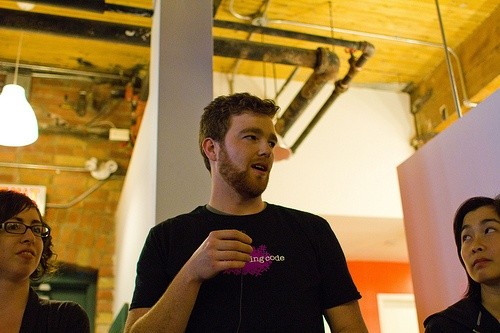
left=125, top=93, right=370, bottom=333
left=423, top=196, right=500, bottom=333
left=0, top=188, right=90, bottom=333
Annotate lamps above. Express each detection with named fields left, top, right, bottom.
left=0, top=30, right=38, bottom=147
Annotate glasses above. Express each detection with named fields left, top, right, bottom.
left=0, top=222, right=51, bottom=238
